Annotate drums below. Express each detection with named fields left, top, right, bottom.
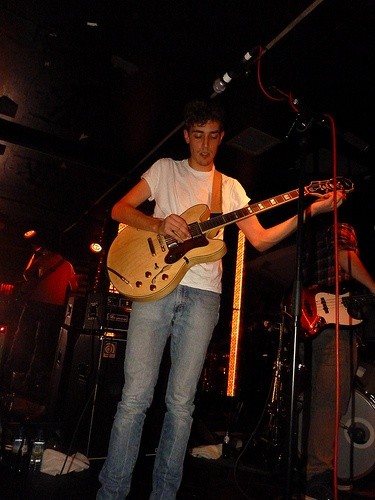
left=196, top=351, right=241, bottom=396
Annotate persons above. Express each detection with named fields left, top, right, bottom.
left=305, top=221, right=375, bottom=500
left=4, top=238, right=80, bottom=390
left=93, top=98, right=346, bottom=500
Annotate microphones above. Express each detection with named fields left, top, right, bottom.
left=212, top=46, right=257, bottom=94
left=341, top=424, right=366, bottom=442
left=31, top=251, right=45, bottom=256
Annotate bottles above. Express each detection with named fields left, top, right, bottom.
left=12, top=427, right=46, bottom=473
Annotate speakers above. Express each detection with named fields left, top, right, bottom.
left=54, top=331, right=172, bottom=461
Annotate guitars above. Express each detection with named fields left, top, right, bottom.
left=279, top=279, right=375, bottom=338
left=105, top=176, right=354, bottom=303
left=11, top=279, right=31, bottom=303
